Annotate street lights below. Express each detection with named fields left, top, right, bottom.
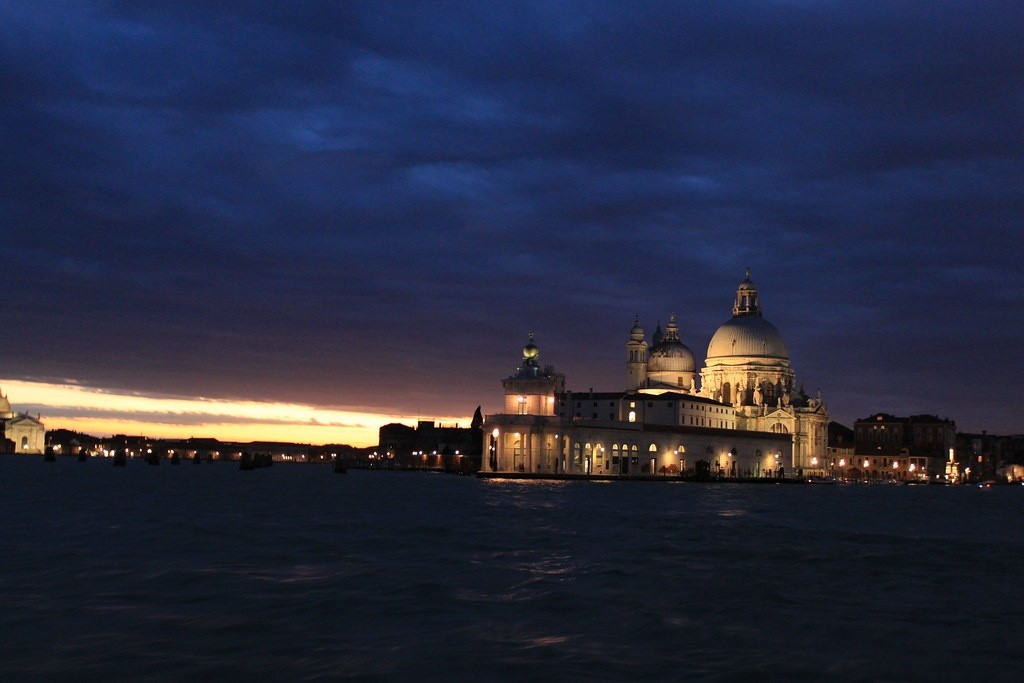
left=893, top=462, right=897, bottom=479
left=493, top=429, right=498, bottom=470
left=812, top=458, right=817, bottom=475
left=840, top=459, right=844, bottom=480
left=864, top=461, right=868, bottom=481
